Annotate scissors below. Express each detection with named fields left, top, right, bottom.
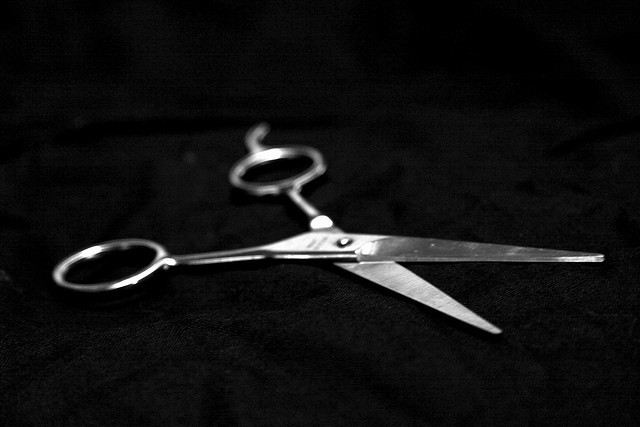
left=52, top=122, right=604, bottom=335
